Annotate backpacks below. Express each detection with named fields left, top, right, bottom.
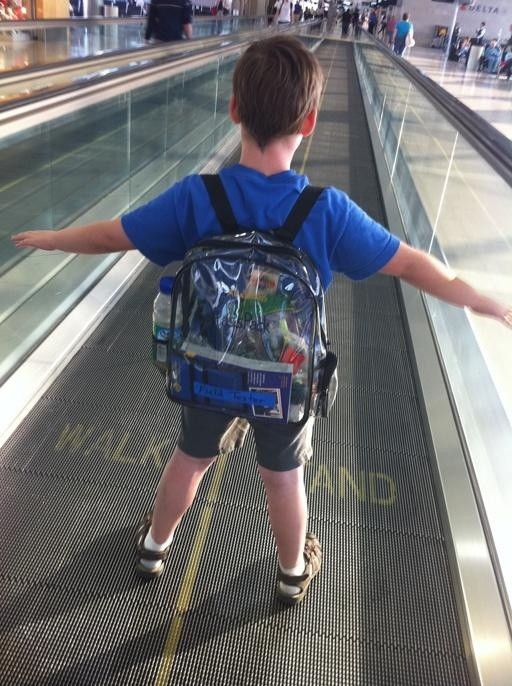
left=146, top=168, right=339, bottom=428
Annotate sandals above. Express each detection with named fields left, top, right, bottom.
left=132, top=508, right=178, bottom=580
left=275, top=529, right=324, bottom=606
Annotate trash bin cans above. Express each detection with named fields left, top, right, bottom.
left=467, top=45, right=484, bottom=73
left=104, top=4, right=118, bottom=18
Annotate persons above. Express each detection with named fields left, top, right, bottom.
left=144, top=0, right=193, bottom=47
left=15, top=34, right=511, bottom=605
left=268, top=1, right=512, bottom=78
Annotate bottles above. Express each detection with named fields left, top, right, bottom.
left=153, top=274, right=182, bottom=373
